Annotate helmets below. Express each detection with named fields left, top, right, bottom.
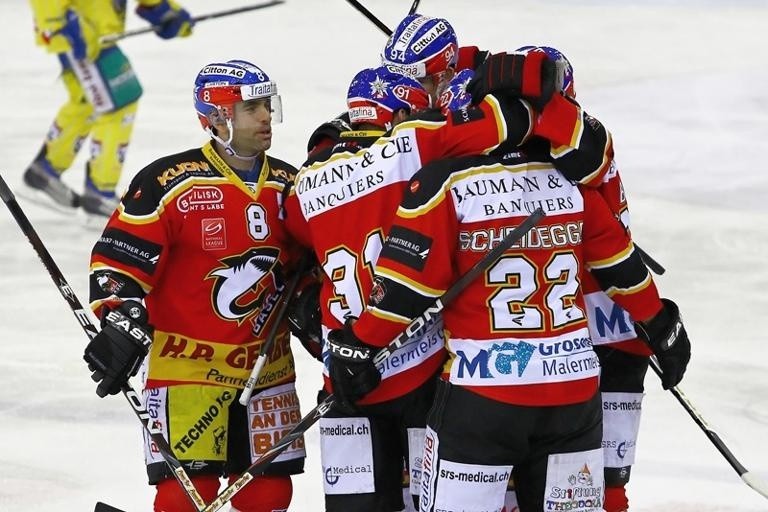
left=348, top=16, right=574, bottom=127
left=193, top=60, right=283, bottom=131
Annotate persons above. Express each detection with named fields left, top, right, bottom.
left=83, top=60, right=325, bottom=512
left=24, top=0, right=196, bottom=218
left=283, top=14, right=691, bottom=512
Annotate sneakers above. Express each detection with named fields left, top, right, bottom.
left=24, top=166, right=119, bottom=216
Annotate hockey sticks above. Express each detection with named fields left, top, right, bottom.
left=95, top=207, right=545, bottom=512
left=646, top=354, right=767, bottom=499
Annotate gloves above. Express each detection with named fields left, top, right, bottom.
left=325, top=328, right=382, bottom=415
left=630, top=298, right=691, bottom=390
left=136, top=1, right=193, bottom=39
left=59, top=10, right=101, bottom=61
left=82, top=301, right=153, bottom=398
